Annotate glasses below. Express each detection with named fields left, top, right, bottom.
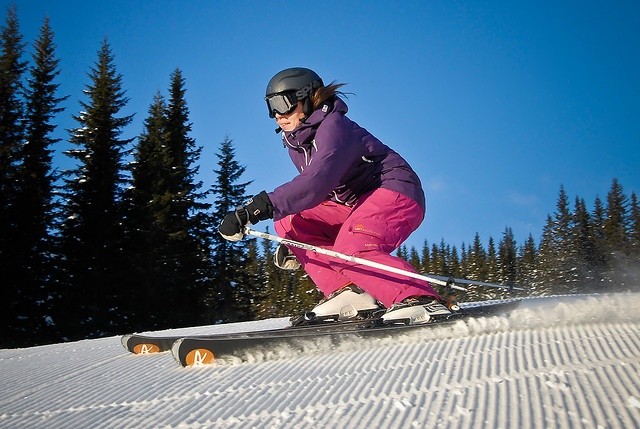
left=265, top=78, right=324, bottom=119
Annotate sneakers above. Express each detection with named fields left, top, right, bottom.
left=306, top=283, right=380, bottom=317
left=381, top=295, right=453, bottom=326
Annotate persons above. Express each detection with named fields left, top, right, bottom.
left=217, top=67, right=455, bottom=325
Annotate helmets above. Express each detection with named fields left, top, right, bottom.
left=264, top=66, right=324, bottom=134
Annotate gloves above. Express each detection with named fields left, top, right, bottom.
left=217, top=189, right=274, bottom=242
left=272, top=243, right=301, bottom=270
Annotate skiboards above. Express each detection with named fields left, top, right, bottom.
left=121, top=295, right=594, bottom=368
